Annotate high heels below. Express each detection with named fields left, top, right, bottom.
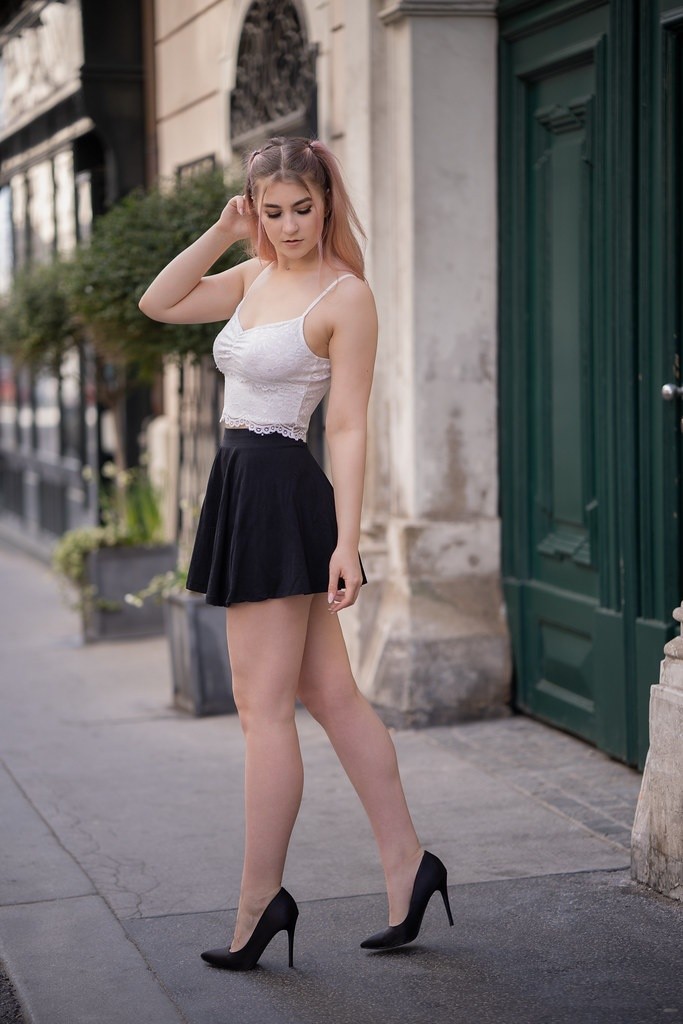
left=200, top=887, right=299, bottom=971
left=360, top=850, right=454, bottom=950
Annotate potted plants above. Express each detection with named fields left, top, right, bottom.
left=51, top=462, right=177, bottom=645
left=125, top=497, right=304, bottom=718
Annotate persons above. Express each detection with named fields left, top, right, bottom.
left=137, top=136, right=455, bottom=968
